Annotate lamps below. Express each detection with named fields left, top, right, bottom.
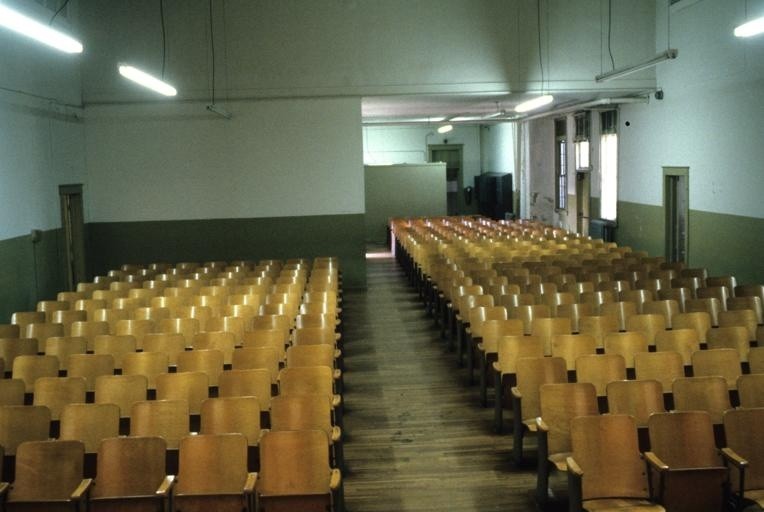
left=595, top=0, right=678, bottom=84
left=205, top=1, right=232, bottom=121
